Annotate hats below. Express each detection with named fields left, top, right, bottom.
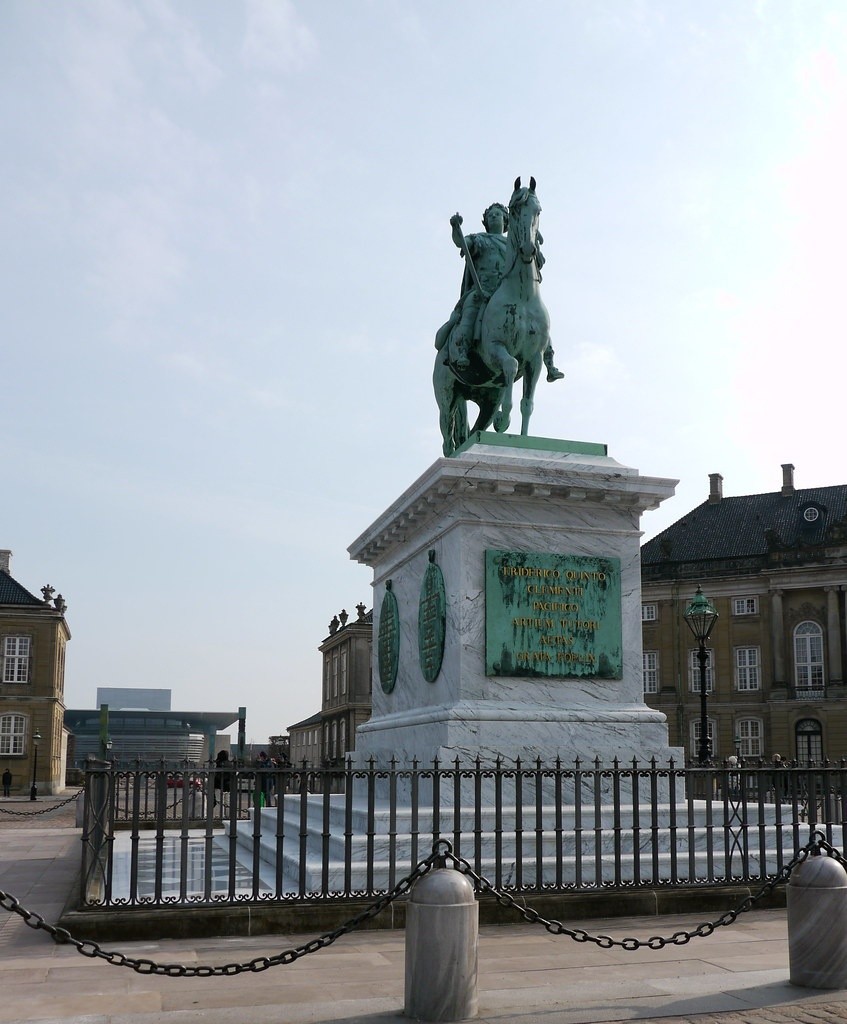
left=259, top=750, right=268, bottom=758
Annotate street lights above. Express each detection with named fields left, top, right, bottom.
left=682, top=583, right=720, bottom=767
left=106, top=737, right=113, bottom=759
left=30, top=728, right=43, bottom=800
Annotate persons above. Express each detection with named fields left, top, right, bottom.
left=214, top=750, right=235, bottom=818
left=772, top=754, right=789, bottom=803
left=728, top=756, right=741, bottom=801
left=256, top=751, right=278, bottom=807
left=450, top=203, right=565, bottom=382
left=2, top=768, right=12, bottom=797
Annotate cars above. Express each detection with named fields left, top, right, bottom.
left=167, top=777, right=198, bottom=788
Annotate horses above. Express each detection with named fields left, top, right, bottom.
left=430, top=176, right=565, bottom=454
